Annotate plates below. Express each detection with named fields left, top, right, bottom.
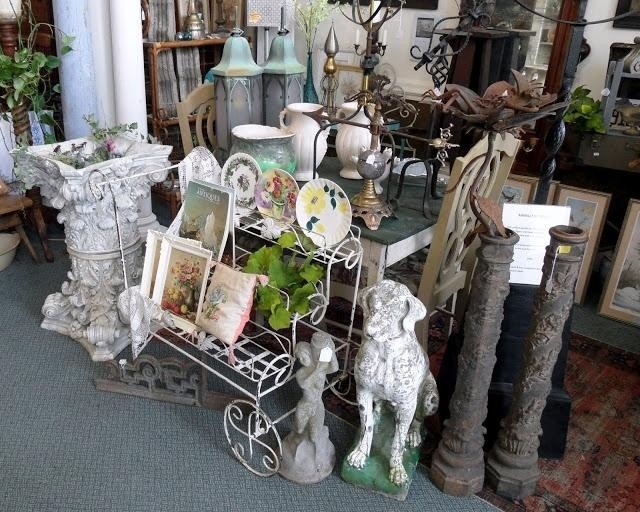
left=253, top=169, right=302, bottom=224
left=296, top=178, right=353, bottom=248
left=220, top=154, right=261, bottom=216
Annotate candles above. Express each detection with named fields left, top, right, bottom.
left=355, top=0, right=388, bottom=47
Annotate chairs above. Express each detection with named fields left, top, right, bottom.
left=177, top=81, right=218, bottom=167
left=305, top=128, right=526, bottom=354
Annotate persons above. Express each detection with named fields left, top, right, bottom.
left=292, top=332, right=341, bottom=442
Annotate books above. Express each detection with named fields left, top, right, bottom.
left=178, top=181, right=232, bottom=261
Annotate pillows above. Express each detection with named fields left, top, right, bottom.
left=194, top=257, right=268, bottom=366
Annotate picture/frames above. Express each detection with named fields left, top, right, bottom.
left=498, top=171, right=638, bottom=325
left=137, top=226, right=215, bottom=335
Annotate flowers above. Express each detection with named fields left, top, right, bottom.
left=290, top=0, right=351, bottom=51
left=50, top=111, right=137, bottom=167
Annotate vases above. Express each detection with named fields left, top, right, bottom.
left=227, top=54, right=384, bottom=182
left=13, top=134, right=173, bottom=361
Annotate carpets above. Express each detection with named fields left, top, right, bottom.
left=322, top=329, right=638, bottom=511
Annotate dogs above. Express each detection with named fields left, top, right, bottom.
left=346, top=278, right=441, bottom=486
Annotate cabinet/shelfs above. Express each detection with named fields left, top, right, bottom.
left=127, top=187, right=365, bottom=479
left=144, top=35, right=258, bottom=208
